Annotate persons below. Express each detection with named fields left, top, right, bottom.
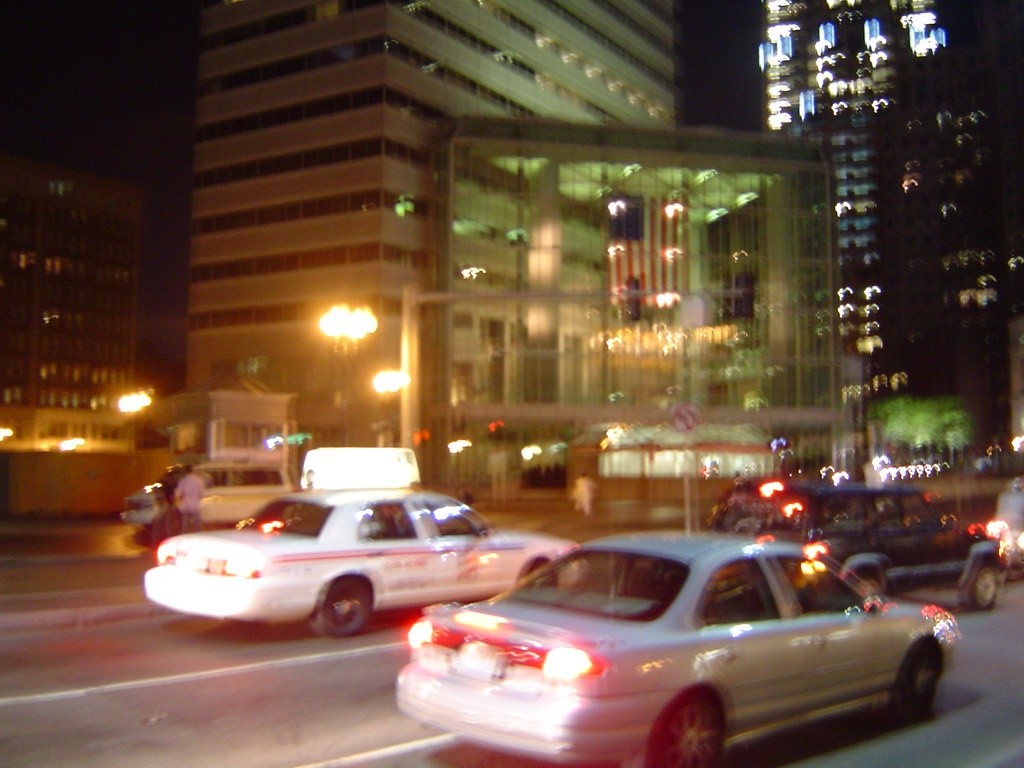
left=149, top=462, right=207, bottom=545
left=568, top=470, right=597, bottom=514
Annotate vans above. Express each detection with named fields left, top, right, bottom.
left=122, top=463, right=294, bottom=525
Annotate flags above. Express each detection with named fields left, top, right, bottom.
left=608, top=190, right=687, bottom=308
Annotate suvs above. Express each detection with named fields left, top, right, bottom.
left=707, top=478, right=1002, bottom=610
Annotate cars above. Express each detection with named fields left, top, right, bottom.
left=142, top=486, right=578, bottom=640
left=395, top=528, right=961, bottom=768
